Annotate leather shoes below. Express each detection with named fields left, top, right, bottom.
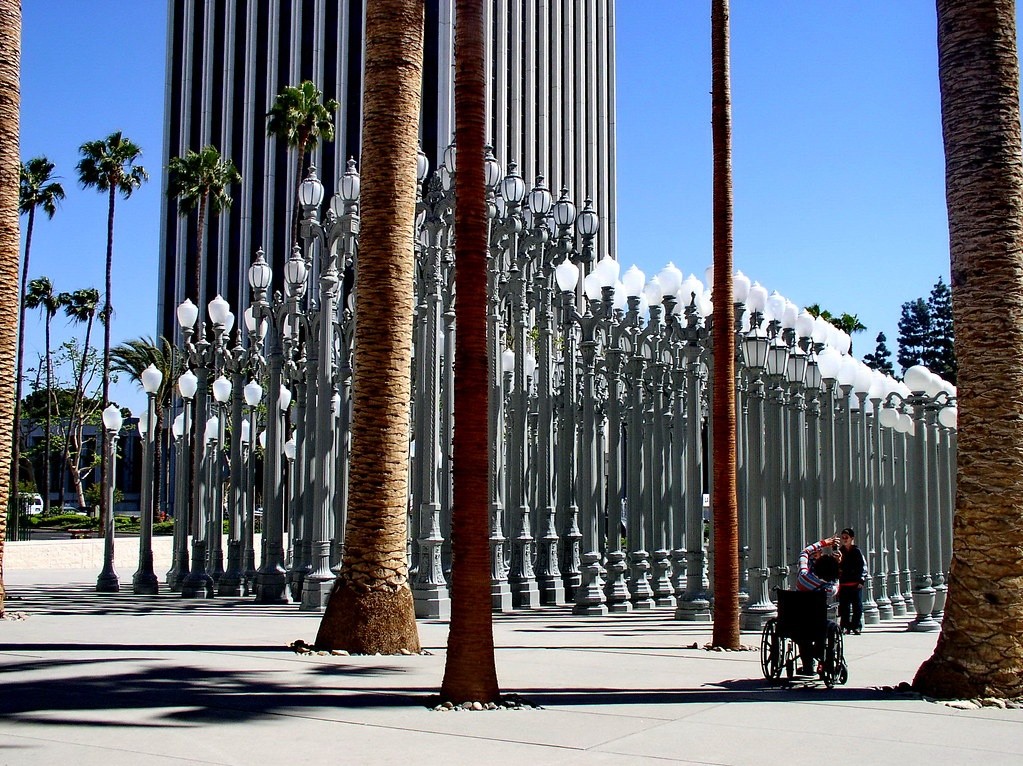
left=852, top=628, right=860, bottom=634
left=841, top=629, right=850, bottom=634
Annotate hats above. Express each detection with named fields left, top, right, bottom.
left=841, top=528, right=855, bottom=538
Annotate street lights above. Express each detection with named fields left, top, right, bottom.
left=96, top=404, right=122, bottom=592
left=133, top=134, right=958, bottom=632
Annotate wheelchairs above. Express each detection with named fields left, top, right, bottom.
left=760, top=584, right=849, bottom=689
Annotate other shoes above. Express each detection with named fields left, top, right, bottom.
left=817, top=664, right=824, bottom=680
left=797, top=667, right=816, bottom=675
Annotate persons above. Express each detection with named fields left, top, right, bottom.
left=839, top=528, right=867, bottom=635
left=797, top=532, right=843, bottom=675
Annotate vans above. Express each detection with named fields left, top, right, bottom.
left=8, top=491, right=44, bottom=515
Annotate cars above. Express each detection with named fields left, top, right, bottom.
left=52, top=506, right=87, bottom=517
